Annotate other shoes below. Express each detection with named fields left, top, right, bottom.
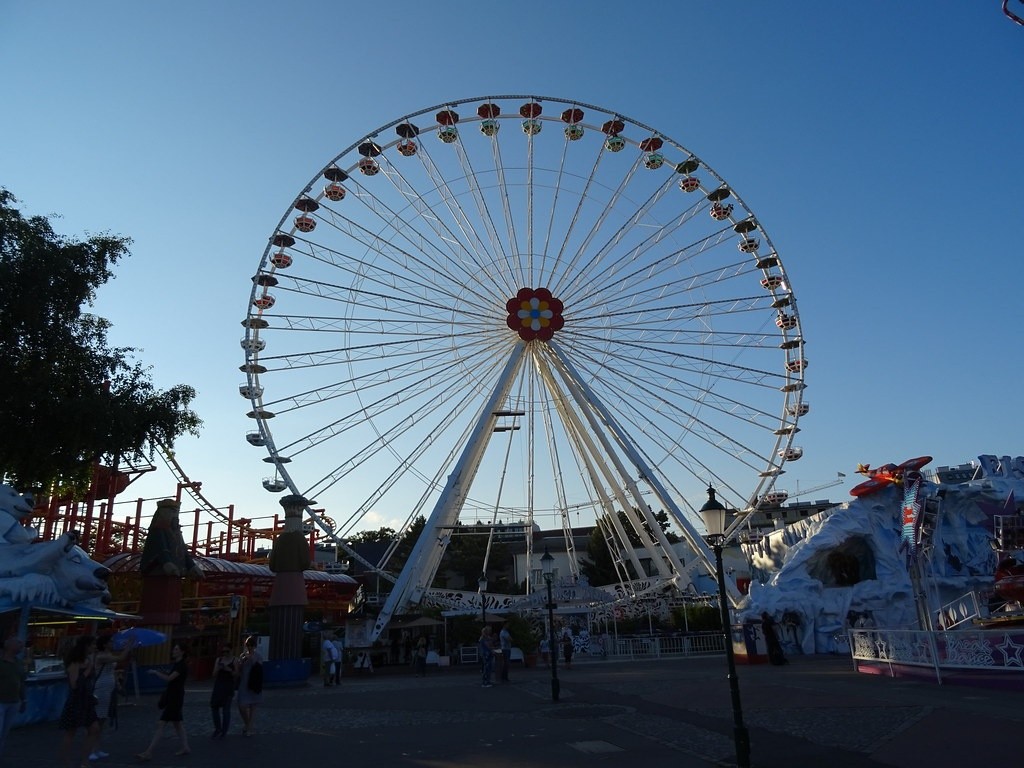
left=220, top=729, right=227, bottom=737
left=88, top=750, right=110, bottom=761
left=135, top=752, right=152, bottom=761
left=213, top=729, right=222, bottom=738
left=176, top=748, right=192, bottom=756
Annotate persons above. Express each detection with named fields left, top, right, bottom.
left=136, top=643, right=194, bottom=760
left=210, top=632, right=263, bottom=740
left=415, top=637, right=427, bottom=677
left=0, top=636, right=27, bottom=759
left=556, top=632, right=574, bottom=665
left=322, top=630, right=343, bottom=687
left=539, top=635, right=551, bottom=668
left=479, top=626, right=497, bottom=688
left=761, top=612, right=789, bottom=666
left=499, top=621, right=514, bottom=683
left=62, top=634, right=135, bottom=768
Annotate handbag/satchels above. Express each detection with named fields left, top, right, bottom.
left=72, top=690, right=99, bottom=710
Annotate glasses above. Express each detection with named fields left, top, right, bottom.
left=247, top=644, right=254, bottom=646
left=222, top=650, right=229, bottom=653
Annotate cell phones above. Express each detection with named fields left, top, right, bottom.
left=219, top=663, right=224, bottom=668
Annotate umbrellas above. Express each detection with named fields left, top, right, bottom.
left=112, top=627, right=166, bottom=697
